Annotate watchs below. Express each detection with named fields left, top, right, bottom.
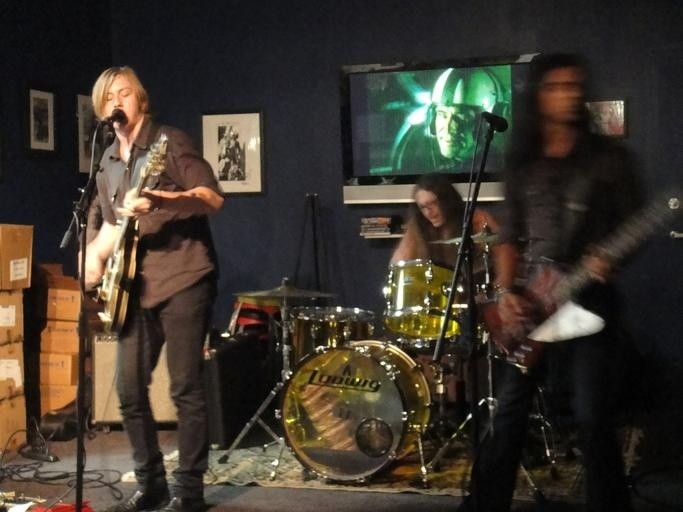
left=151, top=185, right=162, bottom=212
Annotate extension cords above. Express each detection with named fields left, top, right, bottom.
left=19, top=451, right=59, bottom=462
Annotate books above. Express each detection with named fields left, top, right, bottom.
left=355, top=212, right=405, bottom=239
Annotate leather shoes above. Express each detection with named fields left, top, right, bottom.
left=104, top=485, right=171, bottom=512
left=150, top=495, right=205, bottom=512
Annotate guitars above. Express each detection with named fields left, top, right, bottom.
left=88, top=133, right=169, bottom=335
left=480, top=191, right=682, bottom=366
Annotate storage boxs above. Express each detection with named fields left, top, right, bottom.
left=40, top=320, right=80, bottom=355
left=1, top=395, right=28, bottom=454
left=36, top=264, right=66, bottom=277
left=45, top=273, right=84, bottom=323
left=0, top=225, right=35, bottom=290
left=0, top=342, right=26, bottom=400
left=38, top=384, right=81, bottom=419
left=37, top=351, right=80, bottom=385
left=0, top=291, right=25, bottom=347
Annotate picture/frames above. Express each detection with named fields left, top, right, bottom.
left=71, top=92, right=102, bottom=177
left=580, top=96, right=630, bottom=140
left=200, top=108, right=268, bottom=198
left=20, top=81, right=61, bottom=160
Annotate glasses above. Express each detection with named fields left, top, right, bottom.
left=418, top=200, right=437, bottom=211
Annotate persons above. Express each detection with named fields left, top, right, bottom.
left=393, top=67, right=508, bottom=184
left=214, top=124, right=251, bottom=184
left=388, top=177, right=502, bottom=409
left=591, top=102, right=624, bottom=136
left=461, top=52, right=646, bottom=512
left=78, top=66, right=225, bottom=512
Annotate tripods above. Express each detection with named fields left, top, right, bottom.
left=213, top=299, right=305, bottom=479
left=394, top=137, right=492, bottom=489
left=57, top=129, right=124, bottom=512
left=427, top=250, right=541, bottom=504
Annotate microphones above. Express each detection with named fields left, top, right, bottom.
left=104, top=107, right=126, bottom=127
left=478, top=107, right=510, bottom=133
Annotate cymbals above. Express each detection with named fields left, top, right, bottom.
left=430, top=223, right=526, bottom=245
left=234, top=279, right=339, bottom=301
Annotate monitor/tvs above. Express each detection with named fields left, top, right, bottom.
left=338, top=53, right=536, bottom=205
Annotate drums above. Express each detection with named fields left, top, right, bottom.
left=280, top=340, right=432, bottom=484
left=383, top=257, right=466, bottom=340
left=291, top=305, right=376, bottom=369
